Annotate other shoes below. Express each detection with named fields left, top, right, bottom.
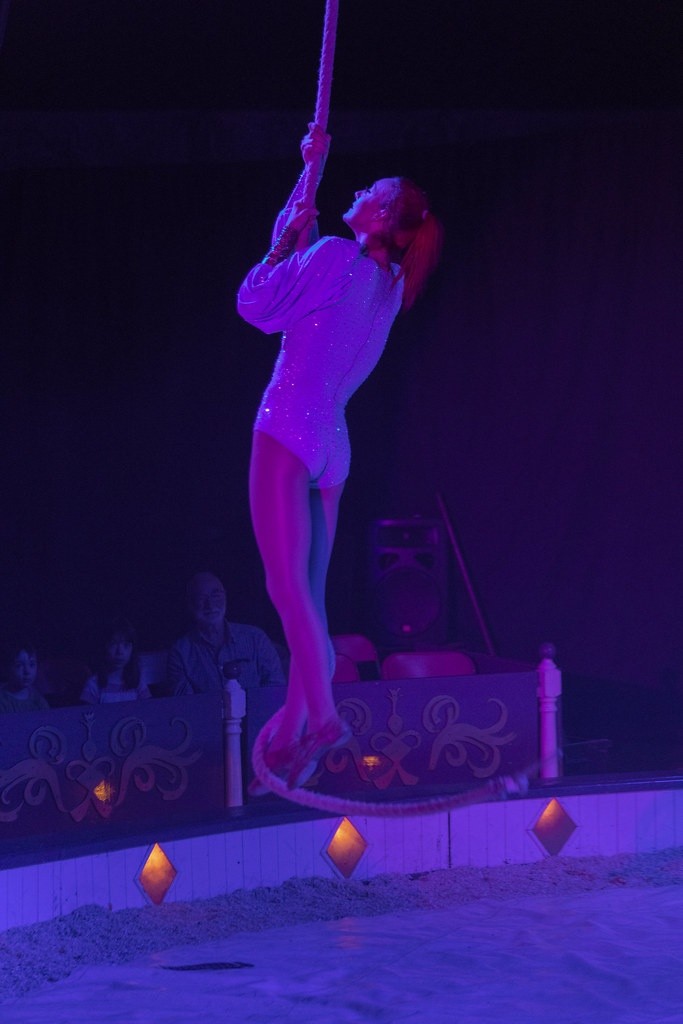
left=248, top=742, right=303, bottom=796
left=285, top=713, right=352, bottom=791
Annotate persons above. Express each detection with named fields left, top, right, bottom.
left=164, top=572, right=284, bottom=698
left=238, top=122, right=446, bottom=794
left=77, top=620, right=152, bottom=707
left=0, top=641, right=52, bottom=714
